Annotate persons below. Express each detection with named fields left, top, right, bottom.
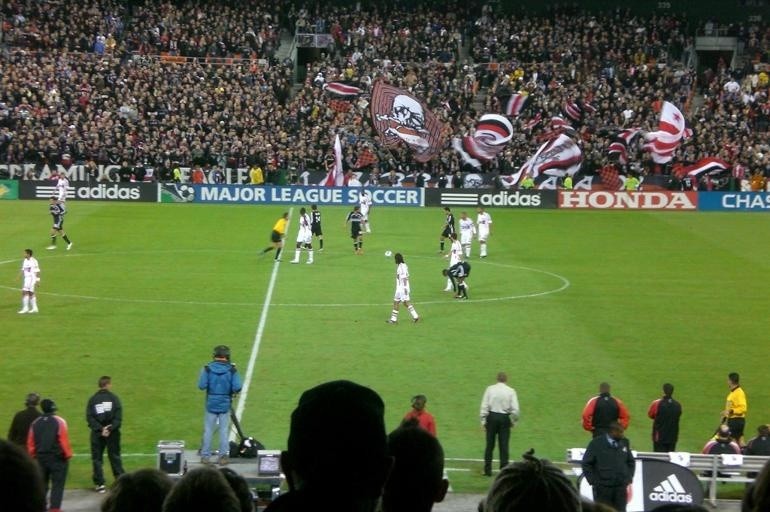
left=17, top=247, right=40, bottom=315
left=443, top=262, right=471, bottom=300
left=442, top=233, right=468, bottom=292
left=0, top=0, right=769, bottom=264
left=1, top=345, right=770, bottom=512
left=385, top=253, right=419, bottom=325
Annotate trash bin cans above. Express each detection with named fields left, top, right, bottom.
left=277, top=169, right=287, bottom=184
left=729, top=178, right=741, bottom=191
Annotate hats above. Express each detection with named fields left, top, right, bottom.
left=482, top=448, right=582, bottom=512
left=288, top=381, right=388, bottom=471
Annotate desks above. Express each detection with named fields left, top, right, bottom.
left=171, top=449, right=284, bottom=501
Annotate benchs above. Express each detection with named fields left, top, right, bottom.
left=563, top=448, right=769, bottom=508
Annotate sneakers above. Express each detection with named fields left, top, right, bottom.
left=95, top=485, right=106, bottom=493
left=201, top=456, right=228, bottom=466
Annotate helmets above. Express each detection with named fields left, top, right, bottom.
left=213, top=346, right=230, bottom=355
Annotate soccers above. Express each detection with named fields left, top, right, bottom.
left=385, top=251, right=392, bottom=257
